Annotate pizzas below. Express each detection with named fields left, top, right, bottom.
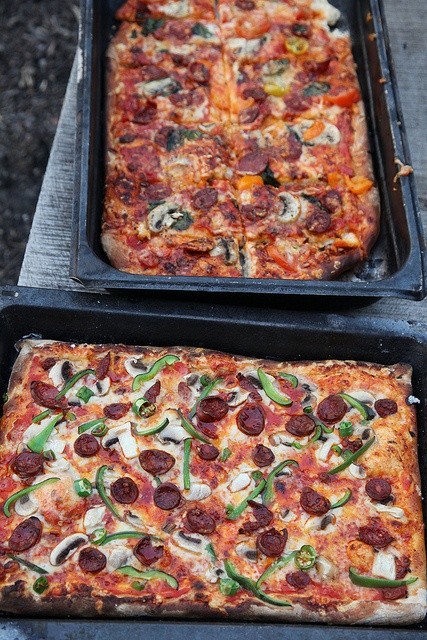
left=0, top=338, right=426, bottom=624
left=101, top=0, right=380, bottom=282
